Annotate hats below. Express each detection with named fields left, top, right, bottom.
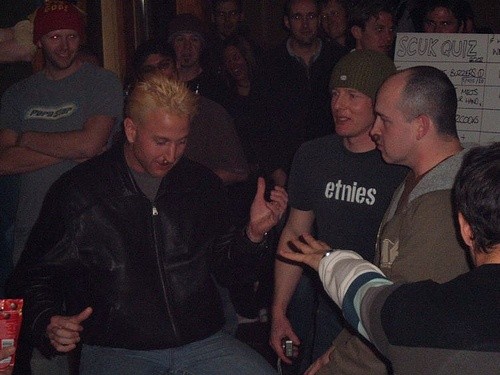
left=167, top=13, right=206, bottom=45
left=32, top=2, right=85, bottom=42
left=329, top=51, right=398, bottom=105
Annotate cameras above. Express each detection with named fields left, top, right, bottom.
left=281, top=337, right=293, bottom=359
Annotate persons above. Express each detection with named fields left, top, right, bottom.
left=0, top=0, right=125, bottom=300
left=15, top=73, right=289, bottom=375
left=409, top=0, right=475, bottom=34
left=319, top=0, right=354, bottom=48
left=259, top=1, right=346, bottom=152
left=129, top=41, right=249, bottom=185
left=303, top=65, right=470, bottom=375
left=280, top=140, right=500, bottom=374
left=161, top=0, right=292, bottom=189
left=347, top=1, right=397, bottom=56
left=269, top=49, right=411, bottom=375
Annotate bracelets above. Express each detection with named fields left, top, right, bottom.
left=16, top=132, right=23, bottom=147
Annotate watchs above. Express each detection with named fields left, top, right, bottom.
left=321, top=249, right=334, bottom=258
